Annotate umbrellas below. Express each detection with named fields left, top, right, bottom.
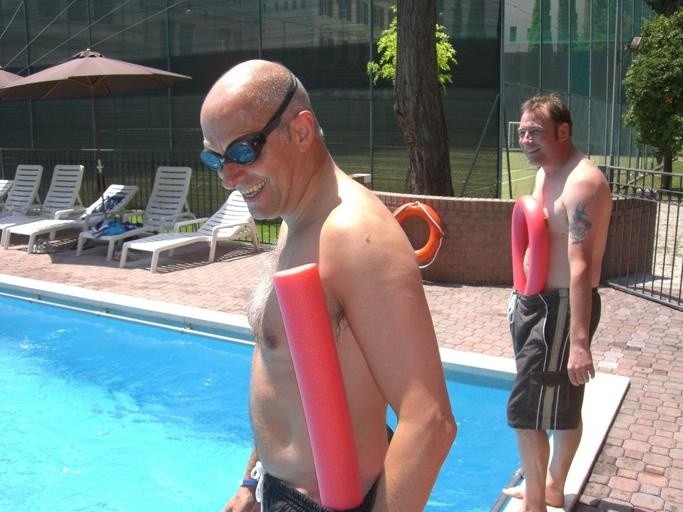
left=0, top=48, right=193, bottom=214
left=1, top=67, right=23, bottom=88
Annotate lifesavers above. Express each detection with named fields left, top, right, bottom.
left=394, top=202, right=441, bottom=263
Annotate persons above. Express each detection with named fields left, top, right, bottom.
left=500, top=94, right=612, bottom=511
left=199, top=58, right=458, bottom=512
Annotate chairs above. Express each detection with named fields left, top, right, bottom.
left=0, top=164, right=259, bottom=273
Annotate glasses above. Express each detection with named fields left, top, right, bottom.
left=200, top=118, right=281, bottom=170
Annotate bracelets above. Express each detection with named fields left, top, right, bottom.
left=240, top=479, right=257, bottom=488
